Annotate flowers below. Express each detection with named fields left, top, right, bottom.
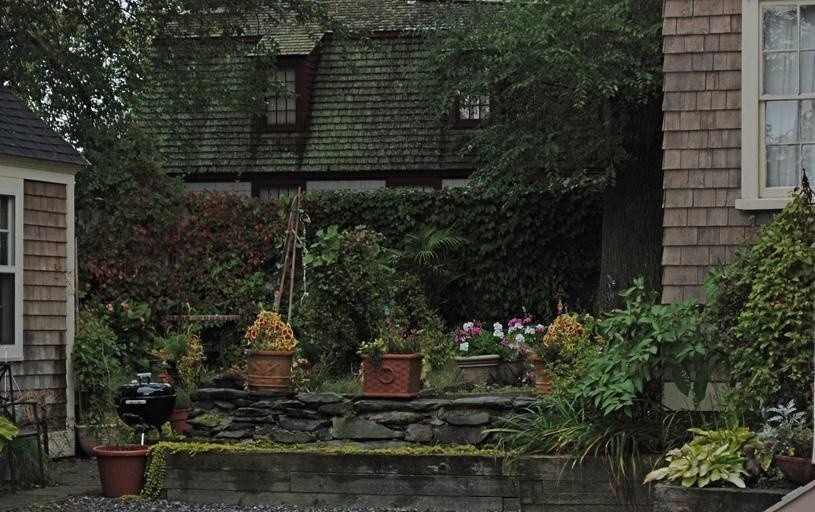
left=357, top=306, right=422, bottom=358
left=446, top=313, right=497, bottom=355
left=244, top=307, right=301, bottom=353
left=491, top=314, right=552, bottom=350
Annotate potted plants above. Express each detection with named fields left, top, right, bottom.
left=642, top=398, right=815, bottom=491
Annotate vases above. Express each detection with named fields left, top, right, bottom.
left=247, top=353, right=300, bottom=394
left=74, top=385, right=149, bottom=497
left=496, top=358, right=532, bottom=388
left=360, top=356, right=429, bottom=397
left=458, top=356, right=500, bottom=391
